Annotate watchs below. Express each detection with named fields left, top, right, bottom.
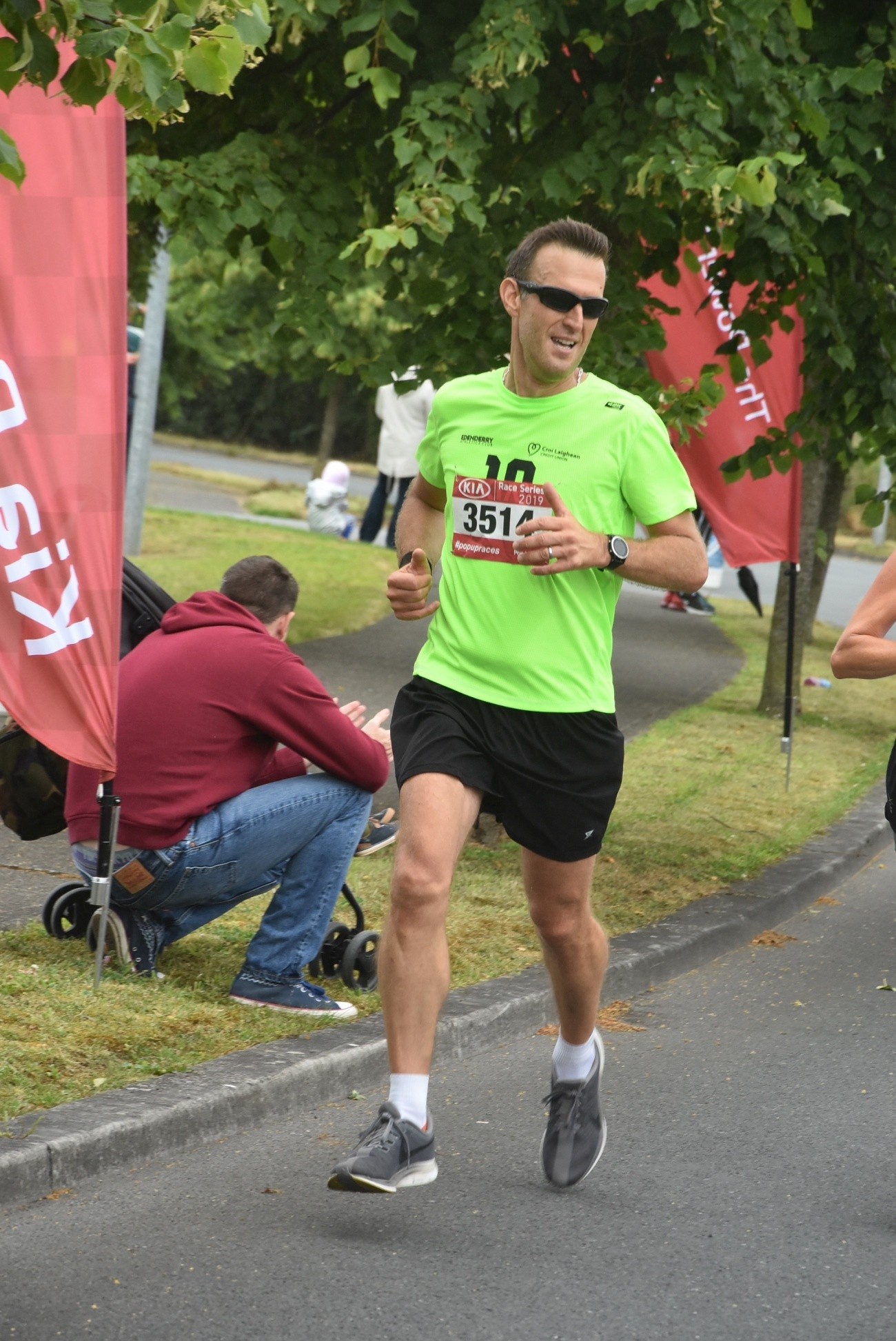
left=597, top=533, right=629, bottom=572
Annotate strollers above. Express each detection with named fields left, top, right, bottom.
left=0, top=557, right=384, bottom=995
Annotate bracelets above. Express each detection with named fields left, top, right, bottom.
left=399, top=551, right=432, bottom=575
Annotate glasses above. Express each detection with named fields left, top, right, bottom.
left=515, top=279, right=608, bottom=319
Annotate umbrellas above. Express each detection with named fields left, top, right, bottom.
left=736, top=565, right=763, bottom=618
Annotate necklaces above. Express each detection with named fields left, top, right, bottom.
left=503, top=364, right=583, bottom=389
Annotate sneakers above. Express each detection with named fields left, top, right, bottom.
left=660, top=589, right=674, bottom=607
left=327, top=1101, right=438, bottom=1194
left=352, top=819, right=400, bottom=857
left=539, top=1025, right=608, bottom=1189
left=227, top=968, right=358, bottom=1019
left=91, top=907, right=156, bottom=980
left=369, top=806, right=395, bottom=824
left=669, top=589, right=715, bottom=617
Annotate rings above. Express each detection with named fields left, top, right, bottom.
left=548, top=546, right=553, bottom=559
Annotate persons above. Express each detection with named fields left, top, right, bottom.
left=325, top=214, right=708, bottom=1195
left=831, top=547, right=896, bottom=854
left=359, top=363, right=435, bottom=549
left=353, top=807, right=400, bottom=857
left=303, top=461, right=356, bottom=542
left=66, top=554, right=392, bottom=1019
left=661, top=499, right=713, bottom=617
